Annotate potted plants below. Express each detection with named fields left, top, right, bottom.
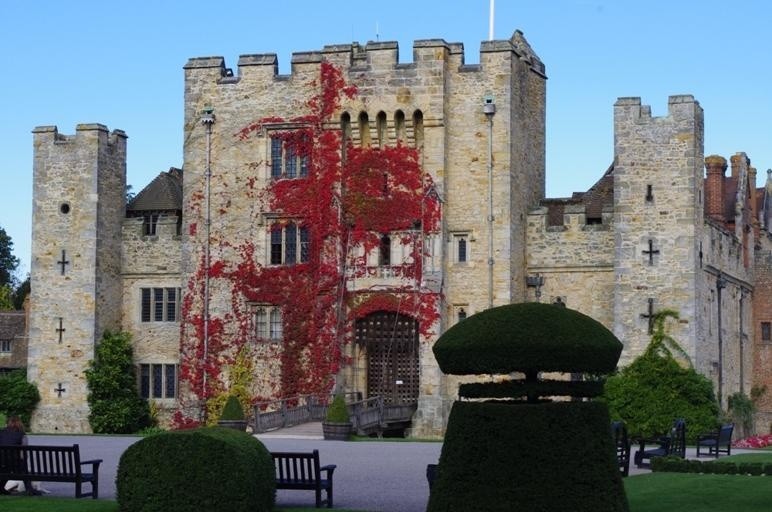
left=217, top=395, right=249, bottom=432
left=322, top=393, right=353, bottom=441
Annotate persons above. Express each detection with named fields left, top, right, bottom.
left=0, top=415, right=43, bottom=495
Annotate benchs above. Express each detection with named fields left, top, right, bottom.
left=0, top=444, right=103, bottom=500
left=634, top=418, right=686, bottom=470
left=612, top=421, right=630, bottom=477
left=696, top=422, right=735, bottom=459
left=269, top=449, right=337, bottom=509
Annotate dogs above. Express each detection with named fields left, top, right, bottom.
left=4, top=480, right=52, bottom=494
